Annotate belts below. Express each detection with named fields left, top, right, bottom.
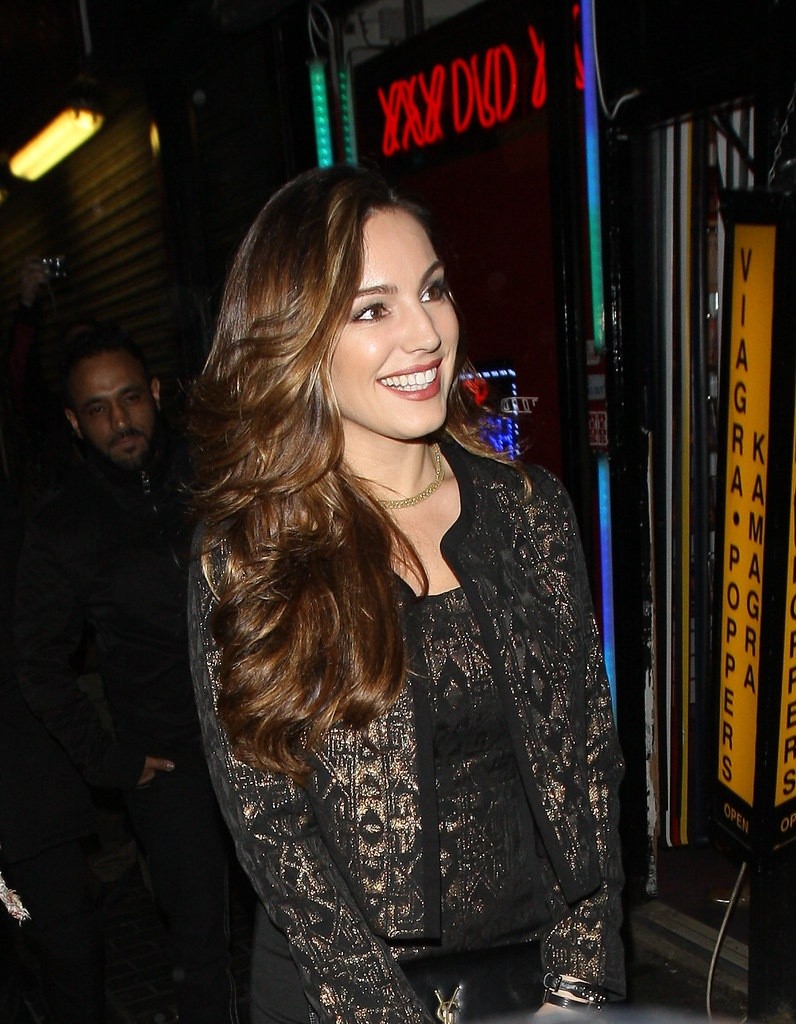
left=396, top=939, right=542, bottom=1024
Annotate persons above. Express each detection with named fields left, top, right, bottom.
left=0, top=327, right=242, bottom=1023
left=187, top=168, right=626, bottom=1024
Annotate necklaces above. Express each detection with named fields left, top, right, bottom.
left=378, top=441, right=444, bottom=509
